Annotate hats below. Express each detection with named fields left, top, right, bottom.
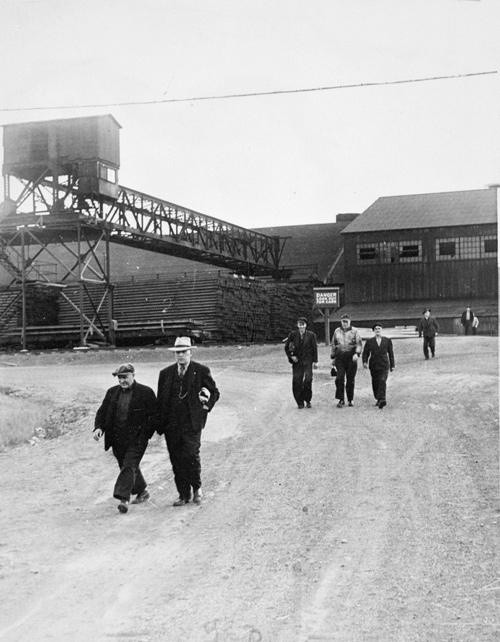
left=423, top=307, right=431, bottom=315
left=298, top=317, right=308, bottom=322
left=112, top=364, right=134, bottom=376
left=341, top=313, right=352, bottom=319
left=168, top=337, right=198, bottom=351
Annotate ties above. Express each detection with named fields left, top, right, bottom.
left=179, top=365, right=185, bottom=374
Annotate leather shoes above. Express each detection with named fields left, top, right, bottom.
left=378, top=399, right=386, bottom=408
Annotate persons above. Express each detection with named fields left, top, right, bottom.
left=156, top=335, right=221, bottom=506
left=91, top=362, right=157, bottom=516
left=363, top=321, right=395, bottom=410
left=471, top=312, right=479, bottom=335
left=460, top=304, right=473, bottom=335
left=284, top=316, right=318, bottom=409
left=418, top=307, right=440, bottom=360
left=330, top=313, right=362, bottom=408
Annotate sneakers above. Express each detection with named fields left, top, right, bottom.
left=338, top=400, right=345, bottom=408
left=298, top=404, right=304, bottom=408
left=306, top=402, right=311, bottom=407
left=174, top=498, right=188, bottom=507
left=193, top=492, right=204, bottom=502
left=118, top=503, right=128, bottom=514
left=349, top=400, right=354, bottom=406
left=131, top=492, right=150, bottom=505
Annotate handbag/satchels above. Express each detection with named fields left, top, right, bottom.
left=331, top=368, right=338, bottom=376
